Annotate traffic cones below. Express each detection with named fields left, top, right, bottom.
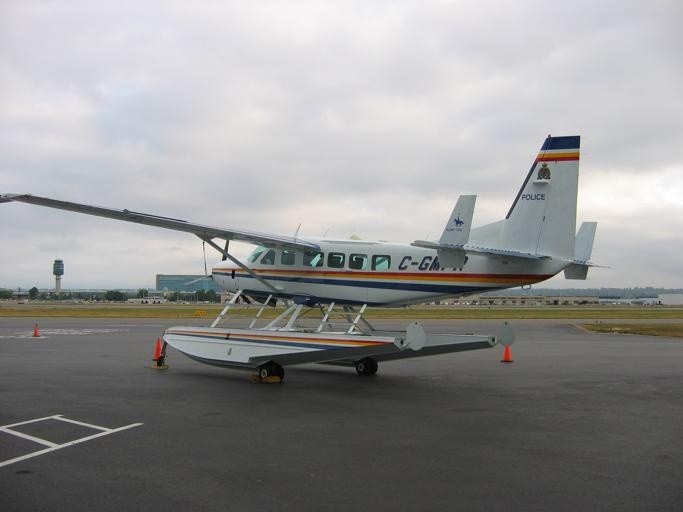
left=32, top=326, right=40, bottom=337
left=151, top=337, right=162, bottom=361
left=500, top=346, right=513, bottom=362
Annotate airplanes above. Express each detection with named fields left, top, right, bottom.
left=0, top=133, right=597, bottom=386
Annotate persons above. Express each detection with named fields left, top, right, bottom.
left=350, top=256, right=359, bottom=267
left=315, top=253, right=323, bottom=268
